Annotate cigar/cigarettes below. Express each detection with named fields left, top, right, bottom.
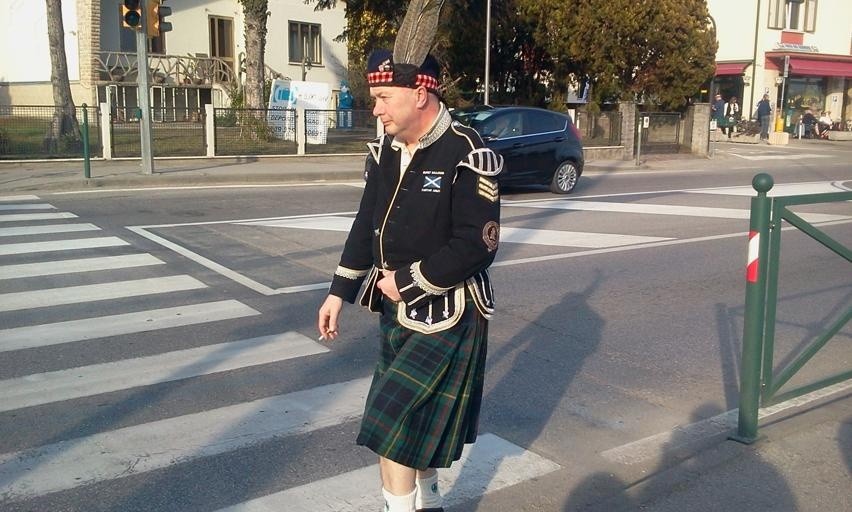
left=319, top=326, right=331, bottom=341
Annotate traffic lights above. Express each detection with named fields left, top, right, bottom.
left=121, top=0, right=171, bottom=39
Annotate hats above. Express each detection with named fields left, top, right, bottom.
left=367, top=49, right=440, bottom=94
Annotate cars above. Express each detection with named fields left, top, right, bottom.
left=364, top=103, right=584, bottom=194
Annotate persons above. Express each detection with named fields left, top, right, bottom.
left=802, top=109, right=830, bottom=138
left=317, top=49, right=505, bottom=512
left=757, top=94, right=766, bottom=132
left=723, top=96, right=739, bottom=138
left=819, top=110, right=839, bottom=131
left=758, top=94, right=771, bottom=142
left=711, top=94, right=726, bottom=131
